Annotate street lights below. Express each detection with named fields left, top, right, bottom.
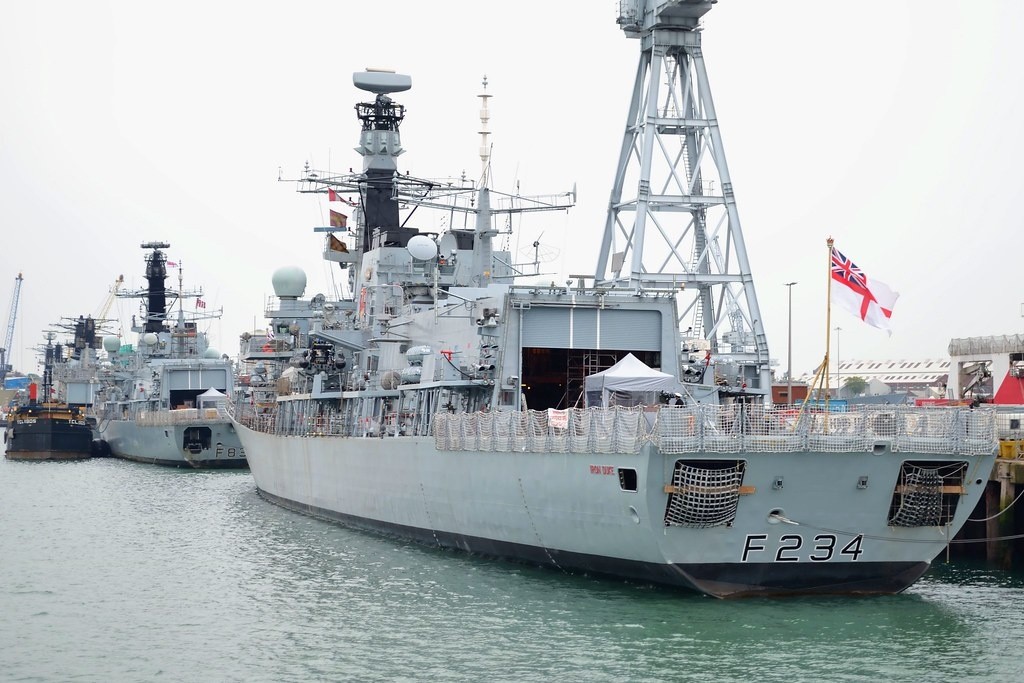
left=782, top=279, right=798, bottom=406
left=833, top=323, right=843, bottom=400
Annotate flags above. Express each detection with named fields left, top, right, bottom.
left=167, top=261, right=177, bottom=268
left=330, top=235, right=346, bottom=253
left=330, top=209, right=347, bottom=227
left=328, top=188, right=346, bottom=202
left=196, top=299, right=205, bottom=309
left=267, top=331, right=276, bottom=339
left=831, top=247, right=900, bottom=339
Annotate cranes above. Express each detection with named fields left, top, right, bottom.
left=0, top=272, right=24, bottom=390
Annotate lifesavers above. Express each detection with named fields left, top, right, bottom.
left=263, top=343, right=273, bottom=353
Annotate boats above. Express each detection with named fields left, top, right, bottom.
left=91, top=239, right=247, bottom=469
left=4, top=314, right=123, bottom=465
left=219, top=0, right=1000, bottom=596
